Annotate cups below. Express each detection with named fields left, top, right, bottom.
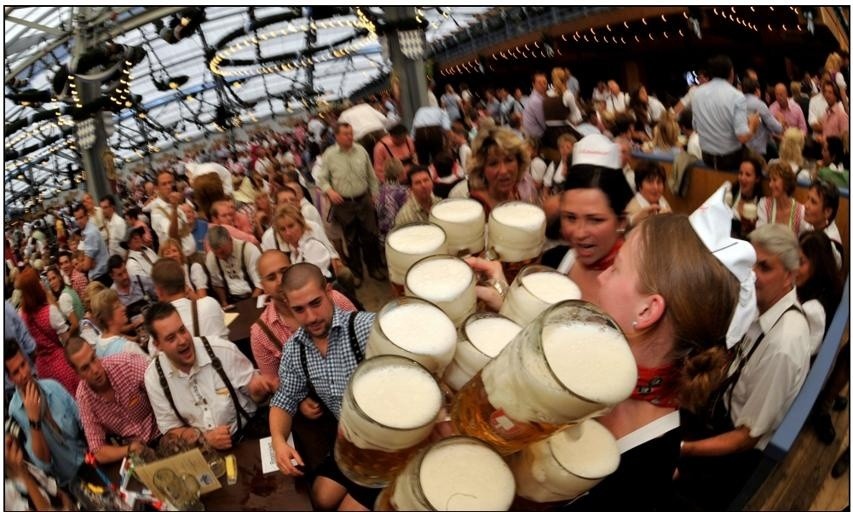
left=428, top=198, right=485, bottom=283
left=482, top=263, right=582, bottom=326
left=127, top=439, right=156, bottom=463
left=132, top=314, right=150, bottom=342
left=365, top=297, right=457, bottom=404
left=741, top=203, right=757, bottom=240
left=404, top=254, right=487, bottom=330
left=444, top=311, right=523, bottom=392
left=159, top=433, right=186, bottom=457
left=512, top=417, right=621, bottom=512
left=179, top=427, right=226, bottom=478
left=375, top=434, right=516, bottom=512
left=383, top=220, right=447, bottom=303
left=486, top=201, right=548, bottom=288
left=451, top=300, right=633, bottom=457
left=152, top=466, right=202, bottom=512
left=334, top=355, right=455, bottom=489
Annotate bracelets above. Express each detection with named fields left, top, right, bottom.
left=679, top=440, right=685, bottom=452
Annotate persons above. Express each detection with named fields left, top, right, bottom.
left=440, top=82, right=526, bottom=137
left=801, top=70, right=821, bottom=95
left=800, top=176, right=844, bottom=276
left=819, top=79, right=850, bottom=170
left=508, top=179, right=759, bottom=512
left=761, top=125, right=815, bottom=188
left=377, top=156, right=410, bottom=236
left=823, top=50, right=851, bottom=112
left=722, top=157, right=763, bottom=240
left=333, top=97, right=398, bottom=168
left=539, top=66, right=584, bottom=152
left=742, top=67, right=759, bottom=83
left=768, top=81, right=810, bottom=143
left=259, top=117, right=325, bottom=223
left=674, top=56, right=766, bottom=175
left=741, top=78, right=785, bottom=163
left=815, top=134, right=845, bottom=176
left=472, top=128, right=544, bottom=286
left=807, top=69, right=848, bottom=161
left=796, top=229, right=847, bottom=370
left=274, top=203, right=336, bottom=290
left=753, top=157, right=807, bottom=239
left=427, top=123, right=474, bottom=185
left=675, top=221, right=811, bottom=459
left=371, top=90, right=397, bottom=119
left=409, top=104, right=452, bottom=169
left=536, top=163, right=636, bottom=304
left=317, top=122, right=387, bottom=289
left=268, top=262, right=388, bottom=512
left=394, top=164, right=444, bottom=228
left=588, top=79, right=684, bottom=170
left=523, top=72, right=549, bottom=150
left=565, top=67, right=581, bottom=103
left=839, top=49, right=851, bottom=101
left=373, top=124, right=421, bottom=185
left=789, top=80, right=811, bottom=123
left=249, top=249, right=359, bottom=420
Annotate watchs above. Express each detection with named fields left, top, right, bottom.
left=28, top=419, right=43, bottom=431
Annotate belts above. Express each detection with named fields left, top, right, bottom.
left=336, top=190, right=369, bottom=202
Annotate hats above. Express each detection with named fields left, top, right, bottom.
left=571, top=135, right=623, bottom=170
left=687, top=186, right=766, bottom=352
left=118, top=225, right=145, bottom=248
